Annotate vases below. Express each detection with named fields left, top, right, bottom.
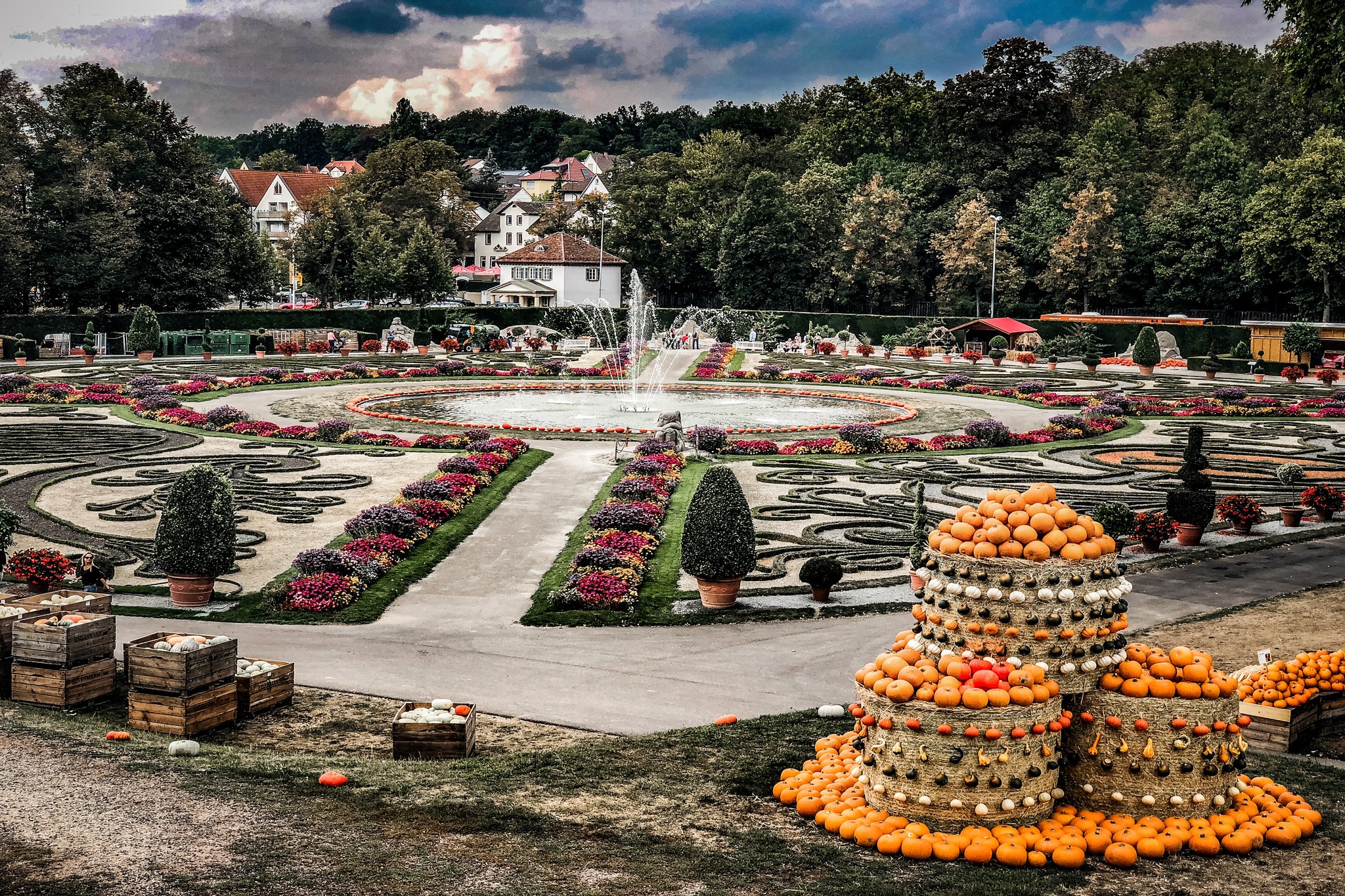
left=532, top=347, right=539, bottom=352
left=1316, top=508, right=1334, bottom=522
left=1022, top=363, right=1029, bottom=369
left=969, top=360, right=976, bottom=365
left=284, top=351, right=292, bottom=356
left=912, top=356, right=920, bottom=361
left=27, top=576, right=49, bottom=594
left=1142, top=536, right=1161, bottom=551
left=1323, top=379, right=1332, bottom=386
left=395, top=349, right=402, bottom=355
left=1288, top=377, right=1296, bottom=383
left=446, top=348, right=453, bottom=354
left=495, top=348, right=501, bottom=353
left=862, top=352, right=869, bottom=358
left=368, top=350, right=376, bottom=356
left=1233, top=521, right=1252, bottom=535
left=824, top=351, right=830, bottom=356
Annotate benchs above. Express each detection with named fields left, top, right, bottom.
left=734, top=340, right=765, bottom=354
left=777, top=341, right=809, bottom=355
left=563, top=339, right=590, bottom=352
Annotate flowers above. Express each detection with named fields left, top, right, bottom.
left=1017, top=353, right=1037, bottom=364
left=440, top=339, right=459, bottom=349
left=962, top=351, right=983, bottom=361
left=4, top=546, right=75, bottom=584
left=1316, top=368, right=1340, bottom=382
left=1128, top=511, right=1182, bottom=541
left=490, top=338, right=509, bottom=349
left=1216, top=494, right=1266, bottom=525
left=817, top=342, right=836, bottom=353
left=389, top=340, right=410, bottom=352
left=361, top=339, right=382, bottom=351
left=525, top=338, right=547, bottom=348
left=857, top=345, right=875, bottom=354
left=1301, top=483, right=1345, bottom=512
left=1281, top=366, right=1304, bottom=379
left=907, top=347, right=927, bottom=357
left=275, top=342, right=300, bottom=355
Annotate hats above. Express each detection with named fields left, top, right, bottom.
left=387, top=330, right=391, bottom=332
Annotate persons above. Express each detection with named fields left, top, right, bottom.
left=804, top=332, right=810, bottom=343
left=523, top=325, right=531, bottom=348
left=791, top=340, right=799, bottom=354
left=783, top=338, right=792, bottom=354
left=845, top=325, right=852, bottom=333
left=748, top=329, right=756, bottom=351
left=662, top=325, right=689, bottom=351
left=460, top=329, right=469, bottom=339
left=327, top=330, right=338, bottom=353
left=692, top=329, right=700, bottom=350
left=1155, top=331, right=1180, bottom=356
left=794, top=332, right=802, bottom=343
left=813, top=332, right=822, bottom=354
left=384, top=330, right=395, bottom=353
left=505, top=328, right=513, bottom=349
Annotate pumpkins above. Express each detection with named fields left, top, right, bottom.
left=848, top=704, right=875, bottom=726
left=1081, top=641, right=1345, bottom=775
left=773, top=730, right=1000, bottom=864
left=992, top=773, right=1322, bottom=868
left=854, top=630, right=1072, bottom=811
left=144, top=634, right=230, bottom=654
left=910, top=482, right=1133, bottom=674
left=398, top=698, right=471, bottom=724
left=818, top=705, right=844, bottom=718
left=235, top=659, right=280, bottom=677
left=0, top=593, right=101, bottom=628
left=716, top=713, right=737, bottom=725
left=854, top=720, right=868, bottom=737
left=106, top=731, right=131, bottom=740
left=319, top=770, right=348, bottom=787
left=169, top=740, right=200, bottom=756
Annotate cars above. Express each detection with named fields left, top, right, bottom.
left=274, top=292, right=520, bottom=309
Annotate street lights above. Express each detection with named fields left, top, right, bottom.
left=596, top=209, right=609, bottom=307
left=988, top=215, right=1002, bottom=318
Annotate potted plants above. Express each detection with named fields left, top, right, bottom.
left=1081, top=334, right=1101, bottom=372
left=340, top=330, right=351, bottom=356
left=1202, top=339, right=1222, bottom=379
left=470, top=327, right=489, bottom=353
left=82, top=322, right=97, bottom=363
left=547, top=332, right=562, bottom=351
left=941, top=330, right=956, bottom=363
left=881, top=335, right=895, bottom=359
left=838, top=331, right=850, bottom=357
left=255, top=328, right=266, bottom=358
left=1253, top=350, right=1265, bottom=382
left=202, top=319, right=213, bottom=360
left=681, top=465, right=757, bottom=608
left=1167, top=489, right=1216, bottom=546
left=512, top=327, right=524, bottom=352
left=128, top=303, right=161, bottom=363
left=413, top=308, right=432, bottom=354
left=1047, top=340, right=1057, bottom=370
left=891, top=333, right=915, bottom=356
left=908, top=482, right=931, bottom=591
left=806, top=345, right=815, bottom=355
left=0, top=498, right=25, bottom=572
left=1276, top=462, right=1306, bottom=527
left=1093, top=502, right=1131, bottom=556
left=1132, top=326, right=1161, bottom=375
left=988, top=335, right=1008, bottom=366
left=14, top=333, right=26, bottom=366
left=154, top=461, right=238, bottom=606
left=799, top=557, right=843, bottom=601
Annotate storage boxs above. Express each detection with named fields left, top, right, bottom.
left=392, top=700, right=476, bottom=758
left=1237, top=651, right=1345, bottom=753
left=1, top=590, right=293, bottom=736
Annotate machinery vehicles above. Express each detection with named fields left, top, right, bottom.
left=444, top=322, right=500, bottom=353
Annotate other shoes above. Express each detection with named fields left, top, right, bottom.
left=384, top=351, right=393, bottom=352
left=326, top=351, right=331, bottom=353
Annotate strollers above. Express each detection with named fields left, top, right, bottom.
left=333, top=337, right=346, bottom=353
